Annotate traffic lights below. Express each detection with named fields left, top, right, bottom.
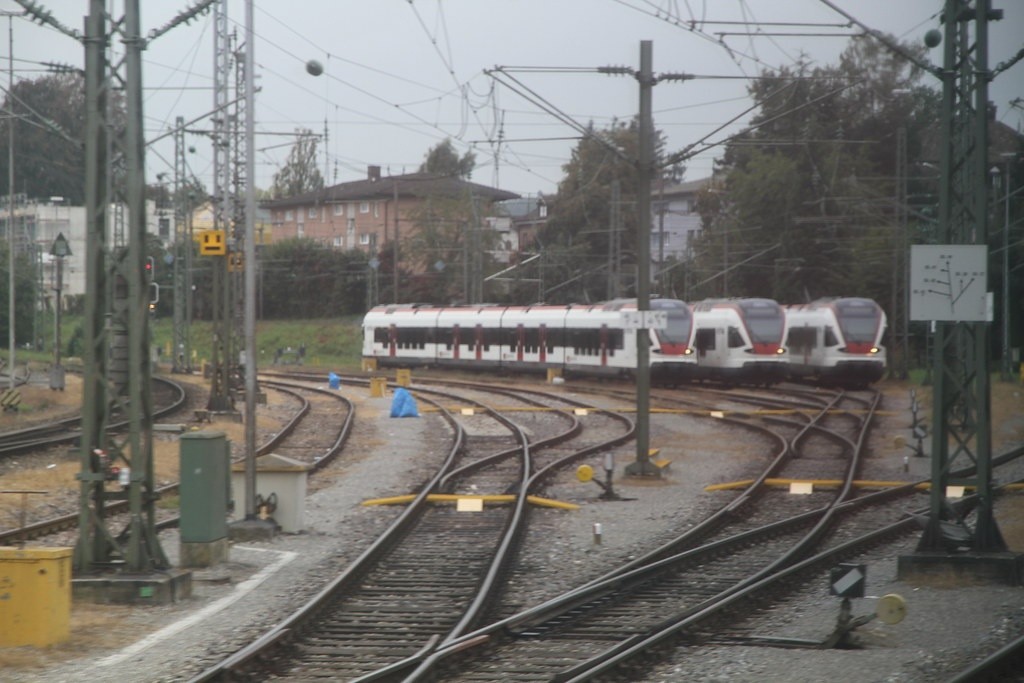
left=150, top=282, right=159, bottom=304
left=146, top=255, right=155, bottom=281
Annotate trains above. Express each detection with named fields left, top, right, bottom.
left=359, top=290, right=698, bottom=386
left=784, top=295, right=889, bottom=388
left=685, top=295, right=793, bottom=386
